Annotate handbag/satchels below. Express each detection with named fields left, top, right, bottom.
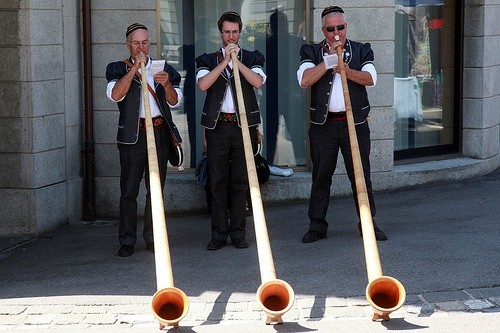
left=228, top=152, right=270, bottom=183
left=196, top=152, right=207, bottom=186
left=169, top=144, right=184, bottom=166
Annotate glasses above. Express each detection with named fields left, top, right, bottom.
left=324, top=25, right=344, bottom=32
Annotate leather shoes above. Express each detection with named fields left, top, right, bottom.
left=207, top=239, right=227, bottom=250
left=231, top=239, right=248, bottom=249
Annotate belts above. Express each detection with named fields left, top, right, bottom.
left=328, top=113, right=346, bottom=118
left=217, top=113, right=238, bottom=124
left=138, top=117, right=167, bottom=128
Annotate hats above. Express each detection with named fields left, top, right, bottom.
left=126, top=23, right=146, bottom=35
left=322, top=7, right=344, bottom=17
left=218, top=11, right=241, bottom=17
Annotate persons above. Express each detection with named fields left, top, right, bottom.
left=106, top=24, right=182, bottom=256
left=297, top=5, right=388, bottom=242
left=195, top=11, right=267, bottom=250
left=255, top=11, right=306, bottom=165
left=178, top=19, right=220, bottom=169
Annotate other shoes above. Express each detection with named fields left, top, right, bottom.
left=146, top=243, right=155, bottom=250
left=302, top=228, right=327, bottom=242
left=118, top=246, right=134, bottom=257
left=358, top=221, right=387, bottom=240
left=245, top=201, right=251, bottom=215
